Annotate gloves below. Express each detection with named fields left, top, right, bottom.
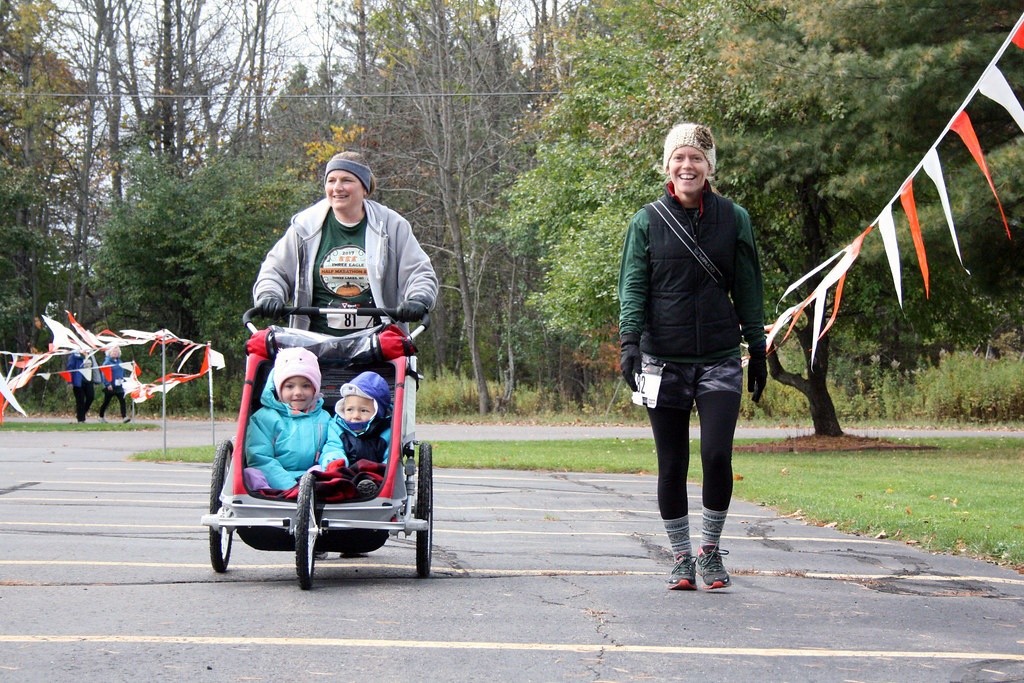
left=748, top=339, right=767, bottom=403
left=391, top=299, right=427, bottom=324
left=260, top=297, right=289, bottom=325
left=621, top=333, right=642, bottom=392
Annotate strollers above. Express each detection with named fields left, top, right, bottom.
left=200, top=306, right=434, bottom=590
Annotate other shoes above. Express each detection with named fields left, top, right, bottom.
left=122, top=416, right=130, bottom=423
left=98, top=417, right=108, bottom=423
left=314, top=552, right=327, bottom=559
left=75, top=413, right=86, bottom=425
left=341, top=551, right=368, bottom=558
left=356, top=478, right=378, bottom=498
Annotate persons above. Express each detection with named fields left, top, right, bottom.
left=98, top=346, right=131, bottom=422
left=252, top=152, right=440, bottom=559
left=243, top=347, right=333, bottom=495
left=64, top=340, right=100, bottom=424
left=319, top=371, right=393, bottom=499
left=616, top=123, right=767, bottom=590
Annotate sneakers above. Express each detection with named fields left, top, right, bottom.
left=668, top=549, right=697, bottom=590
left=695, top=540, right=731, bottom=590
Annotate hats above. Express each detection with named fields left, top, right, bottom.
left=334, top=372, right=391, bottom=438
left=273, top=347, right=322, bottom=410
left=663, top=123, right=716, bottom=176
